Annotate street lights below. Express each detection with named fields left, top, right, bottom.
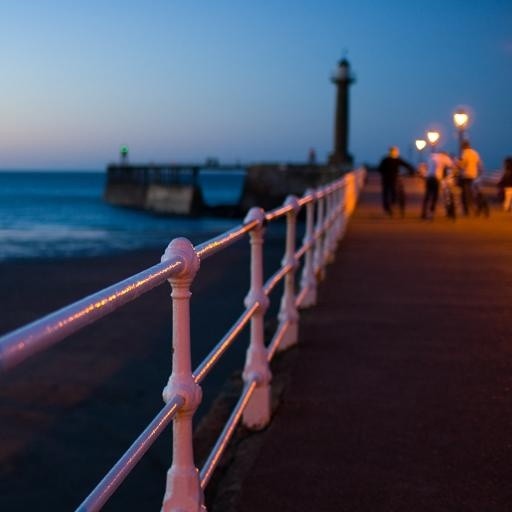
left=415, top=108, right=469, bottom=166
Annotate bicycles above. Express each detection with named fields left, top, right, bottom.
left=396, top=172, right=490, bottom=223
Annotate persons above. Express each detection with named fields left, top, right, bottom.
left=380, top=146, right=415, bottom=215
left=422, top=151, right=454, bottom=218
left=455, top=141, right=481, bottom=216
